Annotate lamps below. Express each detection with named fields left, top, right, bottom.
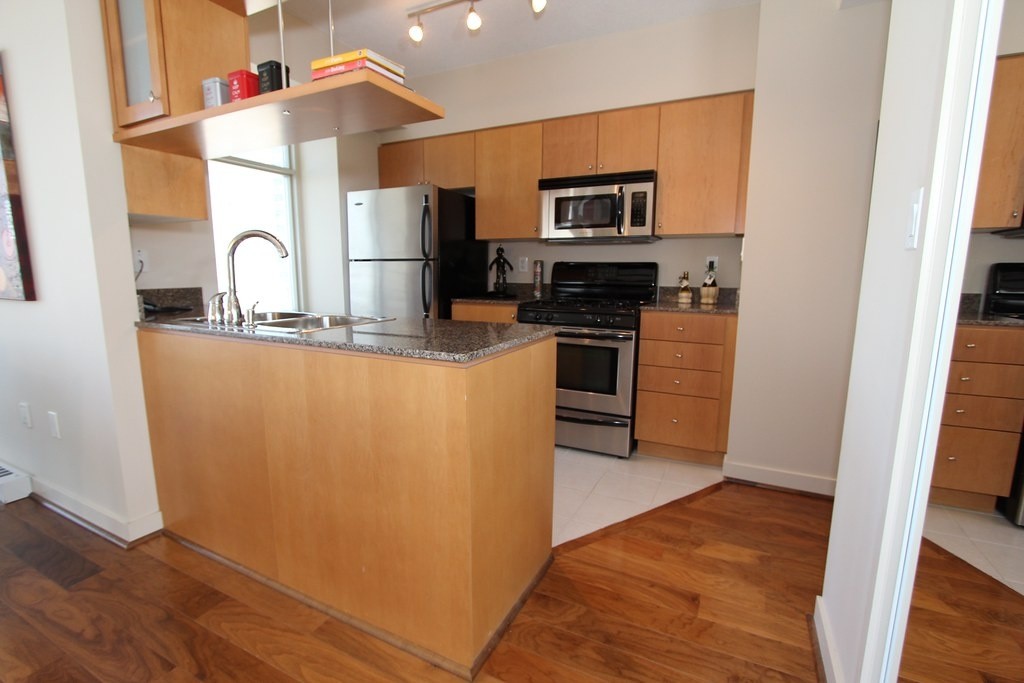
left=409, top=15, right=424, bottom=43
left=467, top=0, right=482, bottom=31
left=531, top=0, right=546, bottom=13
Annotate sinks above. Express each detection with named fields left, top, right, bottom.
left=256, top=314, right=398, bottom=334
left=170, top=309, right=317, bottom=327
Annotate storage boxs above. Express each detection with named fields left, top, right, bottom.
left=971, top=52, right=1023, bottom=233
left=202, top=77, right=229, bottom=110
left=258, top=60, right=290, bottom=94
left=227, top=70, right=259, bottom=102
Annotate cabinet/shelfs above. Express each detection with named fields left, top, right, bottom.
left=634, top=310, right=739, bottom=466
left=100, top=0, right=445, bottom=161
left=377, top=89, right=754, bottom=240
left=929, top=325, right=1024, bottom=512
left=451, top=303, right=518, bottom=323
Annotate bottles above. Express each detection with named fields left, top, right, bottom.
left=533, top=260, right=544, bottom=298
left=701, top=261, right=719, bottom=304
left=678, top=271, right=692, bottom=304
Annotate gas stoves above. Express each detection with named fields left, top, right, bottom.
left=517, top=261, right=659, bottom=329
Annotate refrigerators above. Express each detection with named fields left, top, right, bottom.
left=346, top=184, right=489, bottom=320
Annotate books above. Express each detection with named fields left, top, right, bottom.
left=311, top=48, right=405, bottom=79
left=311, top=58, right=407, bottom=85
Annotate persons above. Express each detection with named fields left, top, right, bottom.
left=488, top=243, right=514, bottom=290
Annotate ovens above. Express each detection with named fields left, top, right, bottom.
left=555, top=327, right=639, bottom=459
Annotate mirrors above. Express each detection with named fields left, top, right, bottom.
left=881, top=0, right=1024, bottom=683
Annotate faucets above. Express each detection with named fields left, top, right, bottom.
left=226, top=229, right=289, bottom=326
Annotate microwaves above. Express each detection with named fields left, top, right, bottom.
left=537, top=169, right=657, bottom=245
